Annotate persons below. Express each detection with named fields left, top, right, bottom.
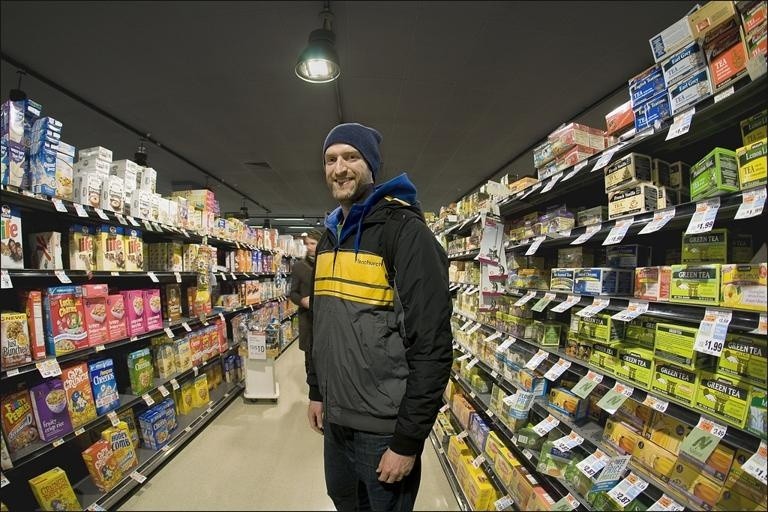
left=308, top=122, right=456, bottom=512
left=288, top=230, right=323, bottom=382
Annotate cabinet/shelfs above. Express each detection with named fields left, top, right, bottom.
left=1, top=182, right=301, bottom=511
left=432, top=51, right=768, bottom=512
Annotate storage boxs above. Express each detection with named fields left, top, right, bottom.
left=650, top=0, right=768, bottom=94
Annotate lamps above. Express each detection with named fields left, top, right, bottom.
left=294, top=10, right=343, bottom=84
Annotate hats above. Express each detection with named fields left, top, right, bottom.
left=324, top=123, right=383, bottom=184
